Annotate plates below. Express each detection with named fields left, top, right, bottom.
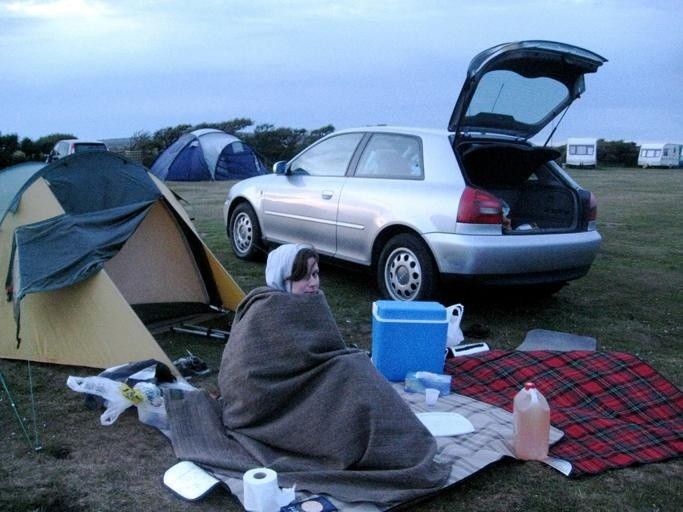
left=415, top=412, right=475, bottom=437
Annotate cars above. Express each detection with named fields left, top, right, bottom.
left=224, top=38, right=608, bottom=302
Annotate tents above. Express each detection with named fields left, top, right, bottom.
left=147, top=125, right=269, bottom=181
left=1, top=152, right=245, bottom=389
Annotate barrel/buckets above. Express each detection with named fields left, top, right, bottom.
left=513, top=382, right=551, bottom=461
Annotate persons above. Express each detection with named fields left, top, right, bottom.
left=217, top=239, right=434, bottom=468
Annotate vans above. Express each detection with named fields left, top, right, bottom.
left=48, top=139, right=107, bottom=160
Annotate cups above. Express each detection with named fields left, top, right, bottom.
left=425, top=388, right=440, bottom=408
